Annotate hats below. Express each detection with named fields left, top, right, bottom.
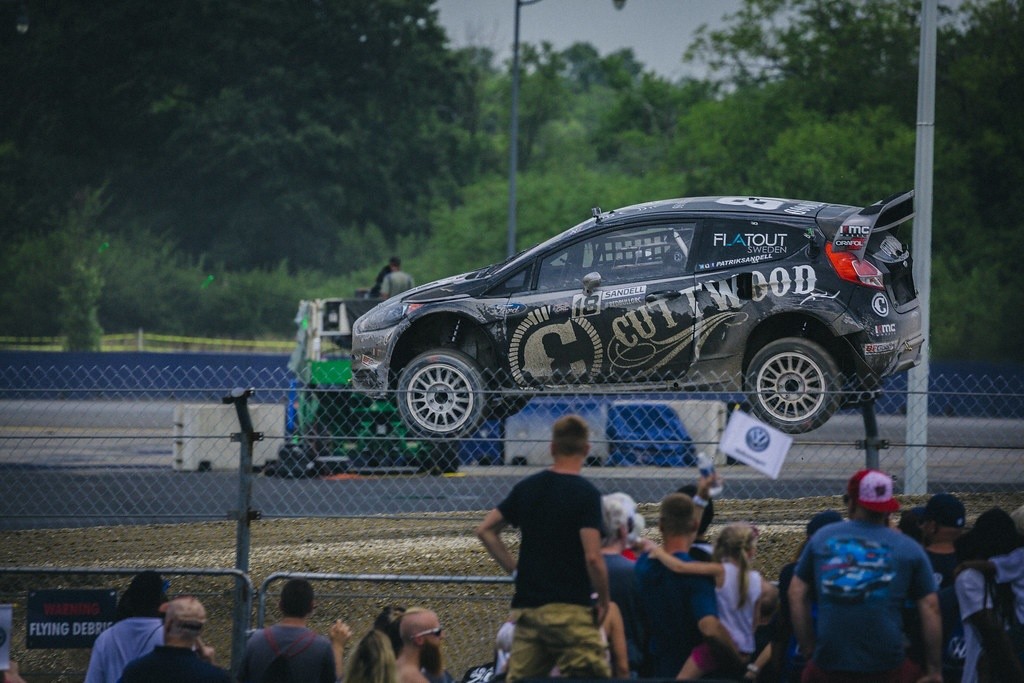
left=807, top=510, right=843, bottom=534
left=159, top=597, right=207, bottom=623
left=912, top=494, right=966, bottom=527
left=849, top=468, right=900, bottom=512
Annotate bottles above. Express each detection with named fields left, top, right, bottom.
left=696, top=452, right=724, bottom=497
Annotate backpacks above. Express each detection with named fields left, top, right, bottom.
left=261, top=627, right=317, bottom=683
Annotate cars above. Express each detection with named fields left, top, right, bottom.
left=351, top=190, right=928, bottom=446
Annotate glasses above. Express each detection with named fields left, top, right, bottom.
left=411, top=628, right=441, bottom=639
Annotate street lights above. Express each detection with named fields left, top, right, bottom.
left=510, top=0, right=625, bottom=259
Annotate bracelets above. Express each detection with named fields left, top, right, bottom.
left=747, top=663, right=759, bottom=673
left=692, top=495, right=708, bottom=508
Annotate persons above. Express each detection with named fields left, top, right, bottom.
left=462, top=622, right=516, bottom=683
left=236, top=579, right=353, bottom=683
left=0, top=628, right=26, bottom=683
left=84, top=570, right=216, bottom=683
left=788, top=466, right=944, bottom=683
left=345, top=631, right=399, bottom=683
left=397, top=606, right=446, bottom=683
left=381, top=254, right=412, bottom=299
left=479, top=413, right=611, bottom=683
left=375, top=604, right=405, bottom=659
left=897, top=493, right=1024, bottom=683
left=115, top=597, right=231, bottom=683
left=587, top=473, right=845, bottom=683
